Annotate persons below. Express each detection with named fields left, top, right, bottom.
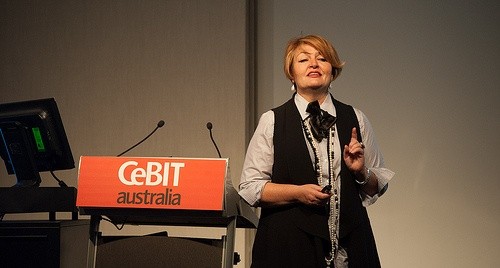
left=238, top=34, right=396, bottom=268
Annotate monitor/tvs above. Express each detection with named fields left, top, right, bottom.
left=0, top=96, right=75, bottom=188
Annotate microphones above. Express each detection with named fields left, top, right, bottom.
left=207, top=122, right=221, bottom=158
left=116, top=121, right=165, bottom=157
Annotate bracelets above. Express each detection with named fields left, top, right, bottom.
left=356, top=169, right=371, bottom=185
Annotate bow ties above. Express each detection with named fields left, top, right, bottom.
left=305, top=99, right=338, bottom=143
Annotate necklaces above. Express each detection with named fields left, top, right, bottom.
left=301, top=117, right=338, bottom=263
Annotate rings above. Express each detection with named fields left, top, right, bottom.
left=360, top=142, right=366, bottom=149
